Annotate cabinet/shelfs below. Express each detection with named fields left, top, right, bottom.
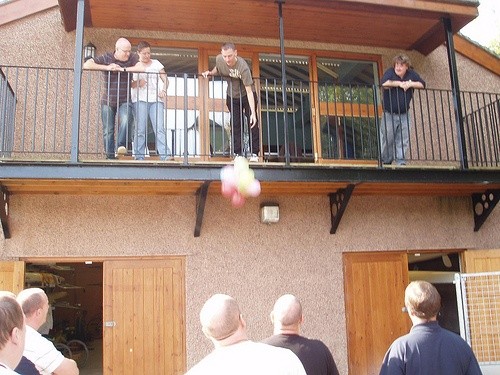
left=25, top=265, right=80, bottom=310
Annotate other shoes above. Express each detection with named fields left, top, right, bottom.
left=114, top=147, right=125, bottom=159
left=250, top=154, right=258, bottom=161
left=396, top=159, right=405, bottom=164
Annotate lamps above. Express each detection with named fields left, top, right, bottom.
left=83, top=41, right=96, bottom=62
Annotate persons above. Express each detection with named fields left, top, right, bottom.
left=131, top=40, right=172, bottom=160
left=82, top=38, right=144, bottom=160
left=202, top=42, right=259, bottom=162
left=380, top=281, right=484, bottom=375
left=380, top=54, right=426, bottom=165
left=15, top=288, right=79, bottom=375
left=0, top=290, right=26, bottom=375
left=13, top=355, right=43, bottom=375
left=185, top=294, right=307, bottom=375
left=259, top=295, right=340, bottom=375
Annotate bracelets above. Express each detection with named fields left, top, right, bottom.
left=162, top=89, right=166, bottom=93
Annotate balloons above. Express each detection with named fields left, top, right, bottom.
left=220, top=156, right=261, bottom=207
